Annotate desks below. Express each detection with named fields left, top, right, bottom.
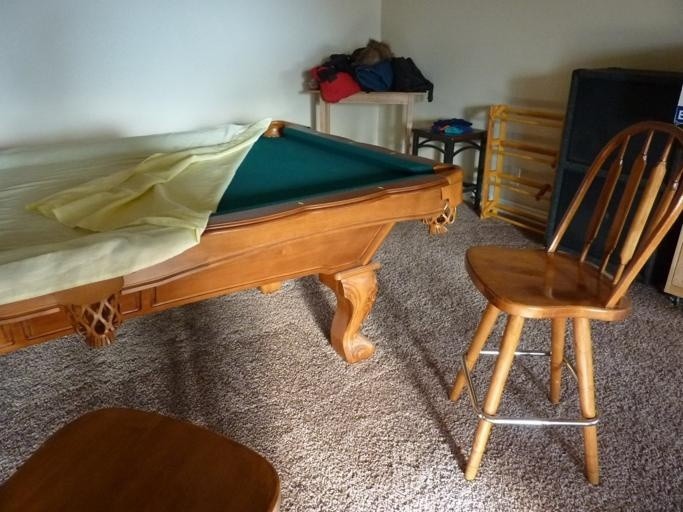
left=308, top=89, right=428, bottom=155
left=0, top=119, right=465, bottom=365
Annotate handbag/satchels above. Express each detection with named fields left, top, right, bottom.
left=311, top=66, right=362, bottom=103
left=392, top=57, right=433, bottom=92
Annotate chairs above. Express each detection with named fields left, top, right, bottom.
left=446, top=118, right=683, bottom=487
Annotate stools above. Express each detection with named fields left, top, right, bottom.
left=1, top=408, right=280, bottom=511
left=412, top=126, right=488, bottom=209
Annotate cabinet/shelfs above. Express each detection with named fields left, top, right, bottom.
left=479, top=103, right=566, bottom=237
left=546, top=67, right=683, bottom=287
